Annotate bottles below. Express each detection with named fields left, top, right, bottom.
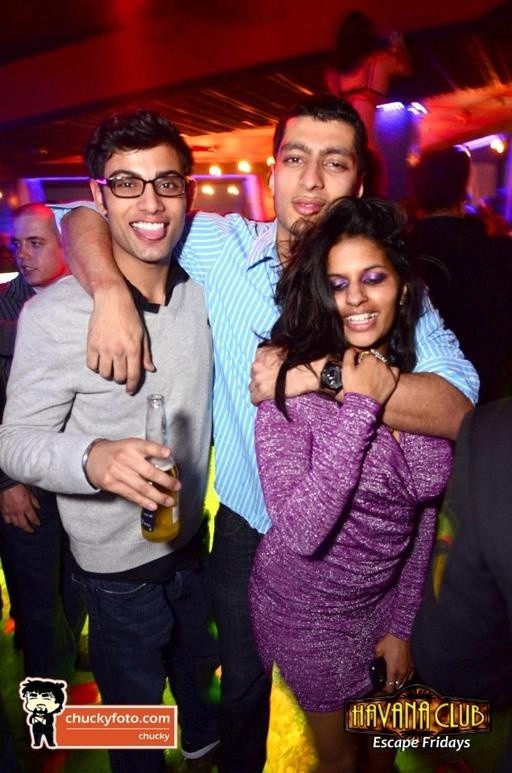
left=141, top=394, right=179, bottom=543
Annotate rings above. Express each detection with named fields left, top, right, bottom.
left=357, top=348, right=388, bottom=361
left=385, top=681, right=398, bottom=685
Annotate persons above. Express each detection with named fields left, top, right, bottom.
left=0, top=198, right=89, bottom=707
left=20, top=677, right=65, bottom=747
left=321, top=16, right=412, bottom=201
left=59, top=88, right=481, bottom=772
left=0, top=110, right=225, bottom=768
left=396, top=142, right=512, bottom=771
left=246, top=196, right=456, bottom=773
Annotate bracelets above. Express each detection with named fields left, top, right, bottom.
left=81, top=438, right=105, bottom=488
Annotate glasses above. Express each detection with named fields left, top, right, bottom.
left=96, top=176, right=190, bottom=198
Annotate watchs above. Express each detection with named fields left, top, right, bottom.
left=319, top=355, right=347, bottom=400
